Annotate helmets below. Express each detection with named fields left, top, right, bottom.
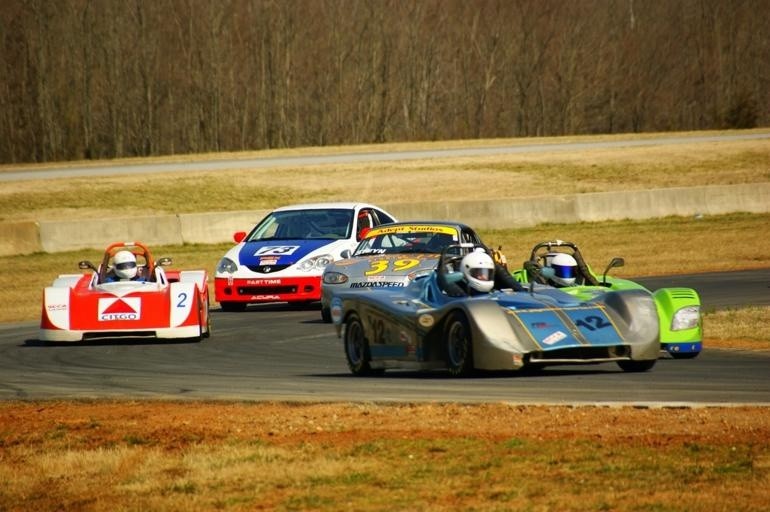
left=550, top=253, right=579, bottom=285
left=112, top=251, right=138, bottom=279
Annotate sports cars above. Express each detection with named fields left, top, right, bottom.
left=214, top=200, right=489, bottom=324
left=39, top=241, right=212, bottom=344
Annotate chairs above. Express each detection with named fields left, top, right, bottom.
left=100, top=242, right=153, bottom=287
left=436, top=240, right=599, bottom=298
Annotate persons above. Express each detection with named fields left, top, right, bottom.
left=548, top=253, right=579, bottom=288
left=105, top=251, right=147, bottom=282
